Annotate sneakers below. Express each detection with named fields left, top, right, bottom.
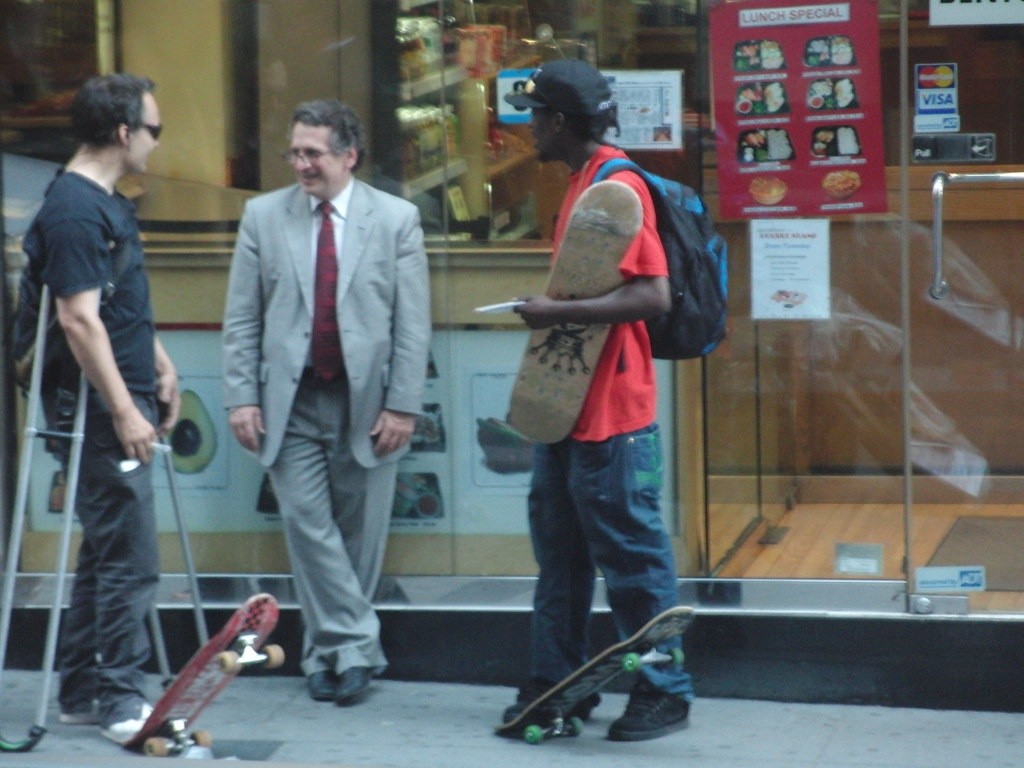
left=608, top=677, right=689, bottom=739
left=502, top=678, right=590, bottom=723
left=99, top=702, right=157, bottom=745
left=60, top=698, right=103, bottom=725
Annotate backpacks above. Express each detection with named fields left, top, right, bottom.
left=590, top=159, right=727, bottom=360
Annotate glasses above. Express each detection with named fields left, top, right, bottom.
left=283, top=149, right=334, bottom=164
left=133, top=123, right=162, bottom=140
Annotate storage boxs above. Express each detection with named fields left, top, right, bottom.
left=493, top=208, right=511, bottom=230
left=444, top=185, right=470, bottom=221
left=458, top=24, right=508, bottom=75
left=492, top=169, right=527, bottom=207
left=394, top=104, right=458, bottom=178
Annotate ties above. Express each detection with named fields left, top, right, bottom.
left=313, top=203, right=341, bottom=381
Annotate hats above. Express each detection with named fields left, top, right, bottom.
left=502, top=60, right=617, bottom=124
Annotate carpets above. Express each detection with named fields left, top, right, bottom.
left=928, top=515, right=1024, bottom=592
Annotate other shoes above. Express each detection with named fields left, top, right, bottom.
left=308, top=672, right=337, bottom=700
left=334, top=666, right=385, bottom=700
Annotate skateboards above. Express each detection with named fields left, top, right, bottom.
left=505, top=179, right=643, bottom=447
left=116, top=590, right=281, bottom=762
left=497, top=603, right=701, bottom=743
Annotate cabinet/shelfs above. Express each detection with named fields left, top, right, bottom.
left=393, top=53, right=549, bottom=243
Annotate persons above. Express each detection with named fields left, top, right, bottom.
left=501, top=54, right=694, bottom=740
left=216, top=97, right=434, bottom=710
left=27, top=68, right=183, bottom=743
